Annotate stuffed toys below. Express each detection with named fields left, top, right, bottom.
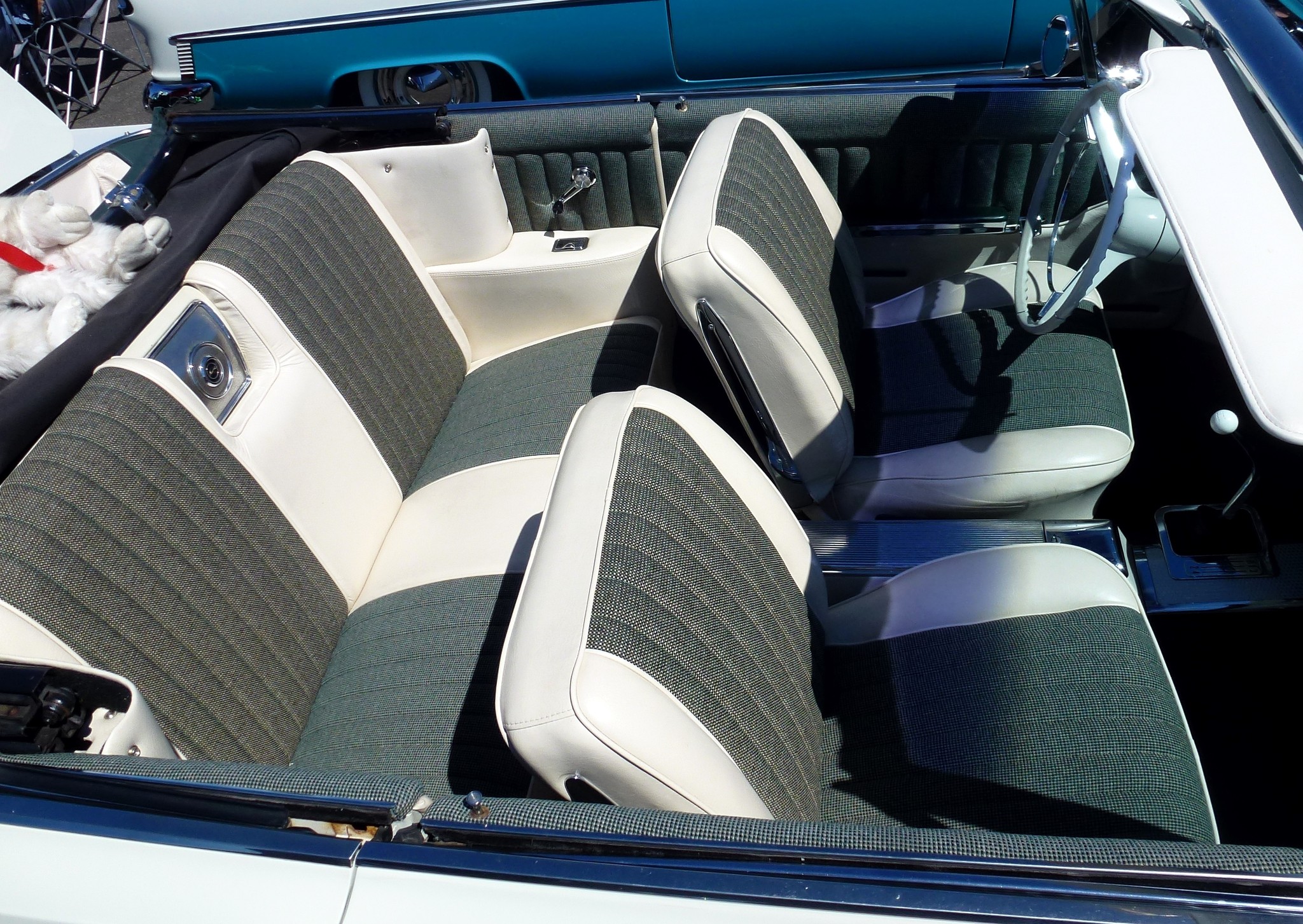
left=0, top=189, right=172, bottom=380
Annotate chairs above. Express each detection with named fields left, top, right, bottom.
left=0, top=0, right=151, bottom=131
left=495, top=387, right=1218, bottom=845
left=655, top=106, right=1135, bottom=525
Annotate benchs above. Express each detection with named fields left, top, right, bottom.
left=0, top=146, right=684, bottom=797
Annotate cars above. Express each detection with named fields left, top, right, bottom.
left=0, top=0, right=1303, bottom=924
